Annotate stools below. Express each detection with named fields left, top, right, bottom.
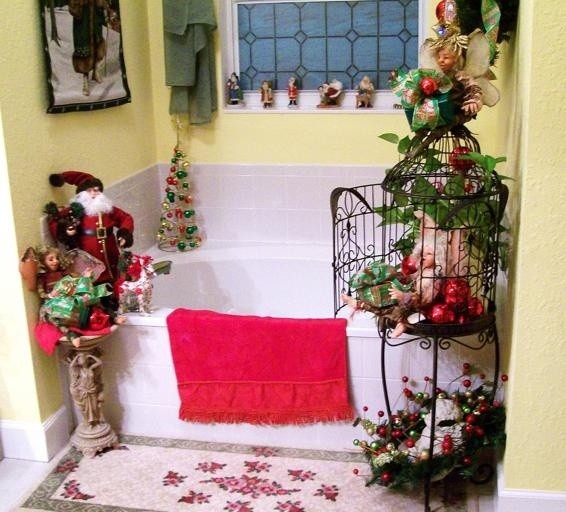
left=380, top=295, right=500, bottom=511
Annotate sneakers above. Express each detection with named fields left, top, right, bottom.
left=92, top=69, right=102, bottom=84
left=82, top=74, right=89, bottom=95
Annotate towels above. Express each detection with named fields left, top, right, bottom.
left=165, top=308, right=354, bottom=424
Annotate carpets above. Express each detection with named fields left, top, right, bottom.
left=10, top=432, right=480, bottom=511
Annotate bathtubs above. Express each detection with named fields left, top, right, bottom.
left=121, top=242, right=406, bottom=340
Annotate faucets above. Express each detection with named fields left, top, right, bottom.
left=152, top=260, right=172, bottom=275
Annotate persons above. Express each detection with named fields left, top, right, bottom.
left=255, top=78, right=273, bottom=109
left=226, top=72, right=240, bottom=104
left=18, top=167, right=156, bottom=349
left=410, top=25, right=484, bottom=134
left=356, top=75, right=375, bottom=108
left=338, top=241, right=453, bottom=340
left=285, top=76, right=299, bottom=106
left=316, top=79, right=345, bottom=108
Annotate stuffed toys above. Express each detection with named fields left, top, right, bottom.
left=406, top=396, right=467, bottom=457
